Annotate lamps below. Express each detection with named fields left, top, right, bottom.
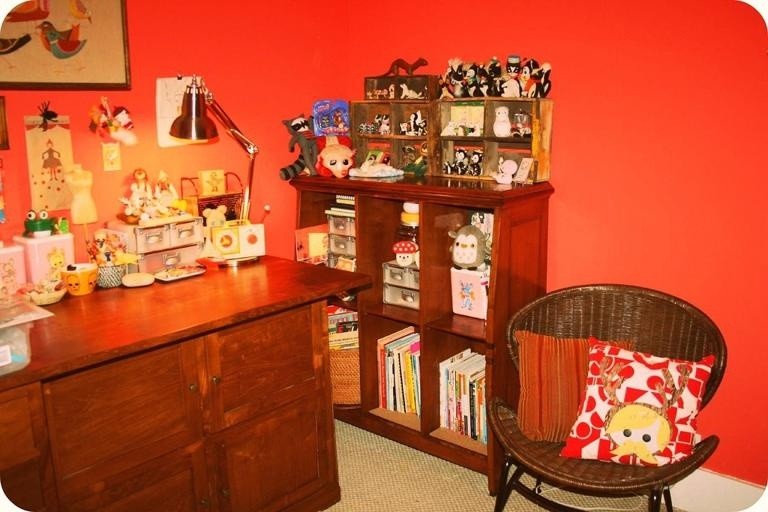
left=170, top=76, right=260, bottom=222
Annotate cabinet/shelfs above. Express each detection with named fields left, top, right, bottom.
left=430, top=94, right=555, bottom=184
left=287, top=173, right=554, bottom=500
left=349, top=99, right=434, bottom=178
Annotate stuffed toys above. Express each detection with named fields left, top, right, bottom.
left=393, top=240, right=418, bottom=266
left=90, top=96, right=138, bottom=146
left=315, top=136, right=356, bottom=179
left=356, top=55, right=552, bottom=185
left=280, top=113, right=318, bottom=183
left=448, top=226, right=490, bottom=271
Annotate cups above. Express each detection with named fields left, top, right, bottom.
left=60, top=263, right=99, bottom=298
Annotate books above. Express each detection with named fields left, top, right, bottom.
left=375, top=325, right=487, bottom=447
left=326, top=196, right=355, bottom=216
left=326, top=306, right=358, bottom=349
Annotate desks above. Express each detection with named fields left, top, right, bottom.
left=0, top=250, right=371, bottom=512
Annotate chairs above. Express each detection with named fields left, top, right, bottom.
left=484, top=284, right=725, bottom=512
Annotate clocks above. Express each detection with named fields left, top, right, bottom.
left=210, top=225, right=241, bottom=257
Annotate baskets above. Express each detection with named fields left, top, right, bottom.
left=329, top=349, right=361, bottom=406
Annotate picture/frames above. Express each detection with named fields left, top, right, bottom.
left=0, top=95, right=11, bottom=151
left=0, top=0, right=132, bottom=91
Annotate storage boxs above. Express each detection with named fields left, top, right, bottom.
left=449, top=265, right=491, bottom=322
left=11, top=233, right=78, bottom=287
left=0, top=322, right=36, bottom=377
left=105, top=213, right=205, bottom=277
left=1, top=242, right=28, bottom=300
left=379, top=259, right=420, bottom=292
left=381, top=284, right=420, bottom=310
left=319, top=203, right=357, bottom=274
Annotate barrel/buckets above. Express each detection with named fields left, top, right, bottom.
left=312, top=97, right=350, bottom=138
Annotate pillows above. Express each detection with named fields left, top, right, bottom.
left=512, top=329, right=629, bottom=442
left=554, top=333, right=714, bottom=469
